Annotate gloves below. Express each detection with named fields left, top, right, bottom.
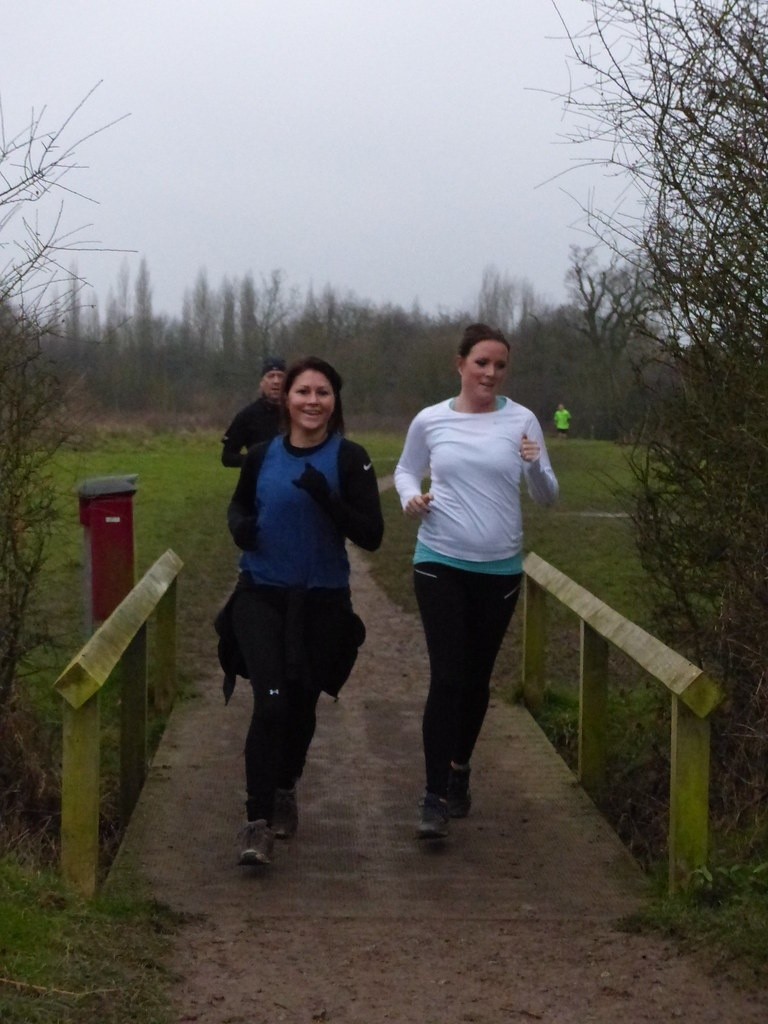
left=232, top=516, right=262, bottom=551
left=291, top=462, right=333, bottom=507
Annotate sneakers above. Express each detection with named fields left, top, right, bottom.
left=449, top=763, right=472, bottom=819
left=416, top=793, right=452, bottom=838
left=273, top=788, right=297, bottom=825
left=236, top=819, right=272, bottom=865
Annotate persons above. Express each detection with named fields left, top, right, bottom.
left=220, top=358, right=290, bottom=468
left=213, top=356, right=383, bottom=863
left=395, top=323, right=558, bottom=837
left=554, top=403, right=572, bottom=438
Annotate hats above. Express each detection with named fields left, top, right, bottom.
left=262, top=360, right=287, bottom=372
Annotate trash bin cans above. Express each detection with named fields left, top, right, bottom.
left=80, top=474, right=138, bottom=624
